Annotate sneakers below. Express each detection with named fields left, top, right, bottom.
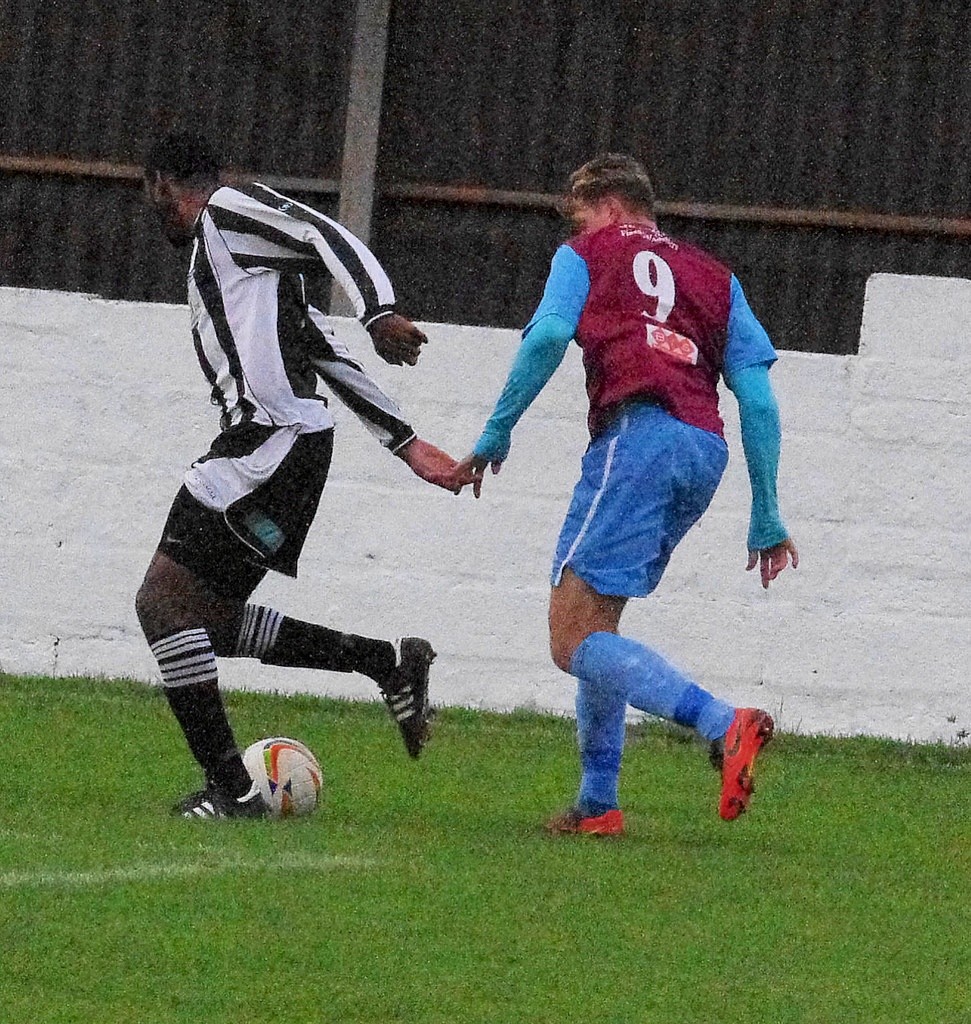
left=708, top=707, right=774, bottom=822
left=546, top=807, right=623, bottom=837
left=169, top=779, right=273, bottom=819
left=377, top=637, right=437, bottom=759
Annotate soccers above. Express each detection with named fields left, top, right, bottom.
left=239, top=736, right=326, bottom=819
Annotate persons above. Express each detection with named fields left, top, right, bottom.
left=142, top=126, right=487, bottom=821
left=445, top=154, right=798, bottom=839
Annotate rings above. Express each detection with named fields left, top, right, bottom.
left=399, top=340, right=405, bottom=353
